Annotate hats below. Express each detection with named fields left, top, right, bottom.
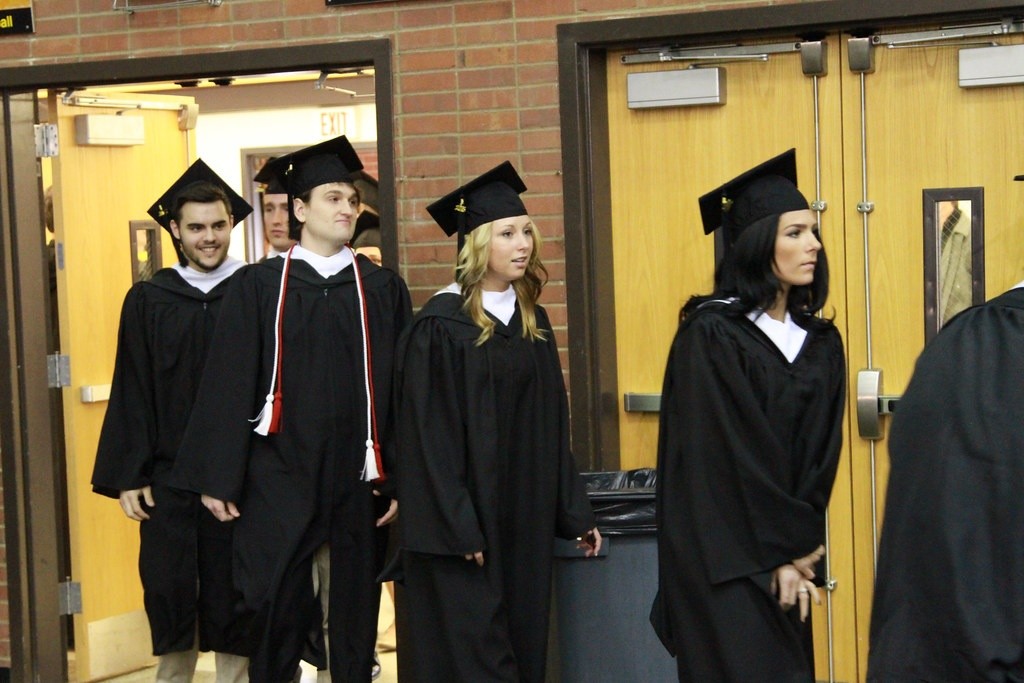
left=349, top=168, right=378, bottom=213
left=426, top=160, right=528, bottom=282
left=349, top=210, right=380, bottom=247
left=271, top=135, right=364, bottom=241
left=253, top=157, right=289, bottom=243
left=146, top=158, right=254, bottom=268
left=697, top=147, right=810, bottom=253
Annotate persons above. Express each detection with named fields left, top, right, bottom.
left=397, top=160, right=601, bottom=683
left=650, top=148, right=846, bottom=683
left=868, top=175, right=1024, bottom=683
left=937, top=200, right=972, bottom=331
left=90, top=134, right=413, bottom=683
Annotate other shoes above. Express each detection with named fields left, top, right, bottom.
left=372, top=656, right=381, bottom=680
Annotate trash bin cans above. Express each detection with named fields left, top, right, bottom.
left=556, top=466, right=679, bottom=683
